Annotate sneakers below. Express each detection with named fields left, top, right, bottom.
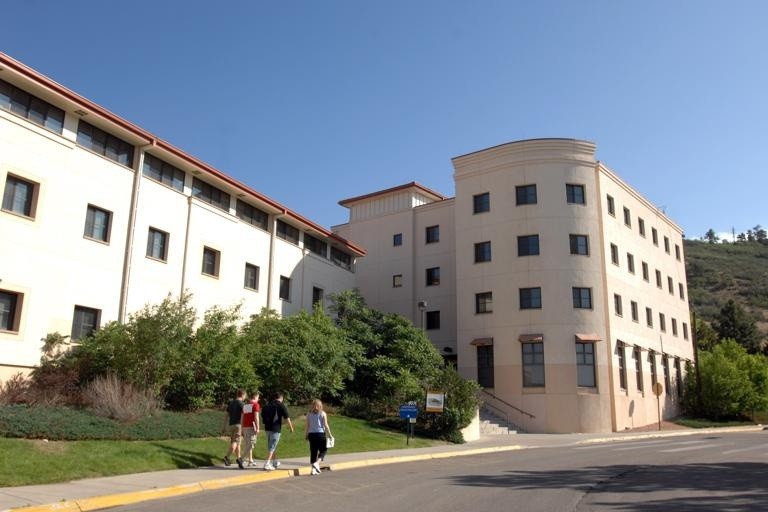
left=310, top=462, right=322, bottom=475
left=274, top=461, right=281, bottom=468
left=247, top=461, right=257, bottom=467
left=223, top=455, right=231, bottom=466
left=235, top=457, right=246, bottom=470
left=263, top=464, right=275, bottom=471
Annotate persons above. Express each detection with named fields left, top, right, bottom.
left=261, top=392, right=293, bottom=470
left=306, top=400, right=333, bottom=475
left=222, top=390, right=244, bottom=467
left=236, top=393, right=259, bottom=469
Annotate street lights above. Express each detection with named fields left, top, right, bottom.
left=418, top=299, right=428, bottom=335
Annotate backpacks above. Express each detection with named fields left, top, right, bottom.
left=261, top=402, right=279, bottom=424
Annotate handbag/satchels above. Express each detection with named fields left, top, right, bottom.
left=325, top=437, right=335, bottom=449
